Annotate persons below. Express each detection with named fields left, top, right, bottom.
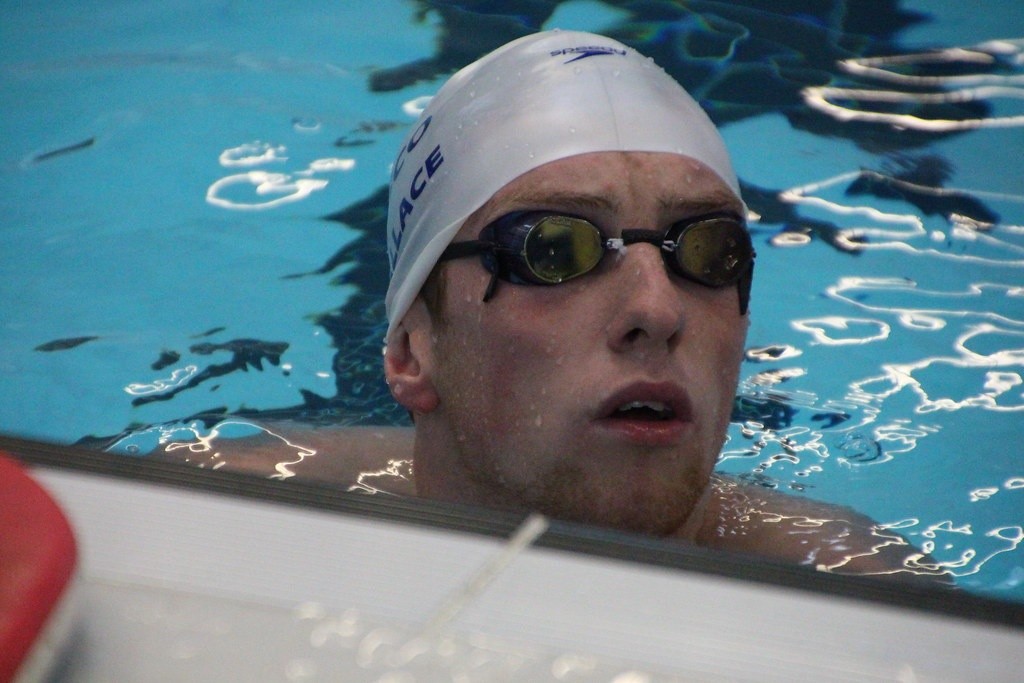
left=382, top=24, right=754, bottom=537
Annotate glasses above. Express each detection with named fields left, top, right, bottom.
left=442, top=208, right=757, bottom=292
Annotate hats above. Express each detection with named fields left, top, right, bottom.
left=382, top=29, right=747, bottom=342
